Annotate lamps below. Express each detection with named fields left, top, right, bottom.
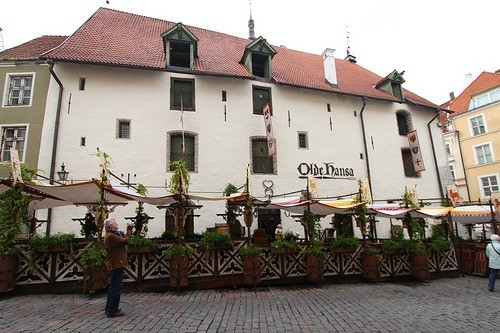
left=57, top=162, right=69, bottom=180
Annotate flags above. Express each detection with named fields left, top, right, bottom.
left=407, top=130, right=425, bottom=173
left=263, top=102, right=275, bottom=158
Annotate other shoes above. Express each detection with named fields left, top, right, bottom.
left=106, top=310, right=125, bottom=318
left=104, top=308, right=121, bottom=314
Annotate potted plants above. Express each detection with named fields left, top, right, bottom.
left=0, top=188, right=22, bottom=293
left=305, top=250, right=327, bottom=282
left=200, top=231, right=234, bottom=262
left=404, top=239, right=429, bottom=282
left=81, top=248, right=108, bottom=293
left=167, top=245, right=188, bottom=286
left=332, top=237, right=358, bottom=252
left=385, top=241, right=404, bottom=254
left=362, top=248, right=380, bottom=279
left=272, top=240, right=298, bottom=254
left=127, top=235, right=156, bottom=254
left=28, top=234, right=75, bottom=271
left=239, top=245, right=261, bottom=285
left=431, top=241, right=451, bottom=250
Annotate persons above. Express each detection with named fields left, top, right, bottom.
left=104, top=219, right=134, bottom=318
left=275, top=224, right=283, bottom=241
left=486, top=234, right=500, bottom=291
